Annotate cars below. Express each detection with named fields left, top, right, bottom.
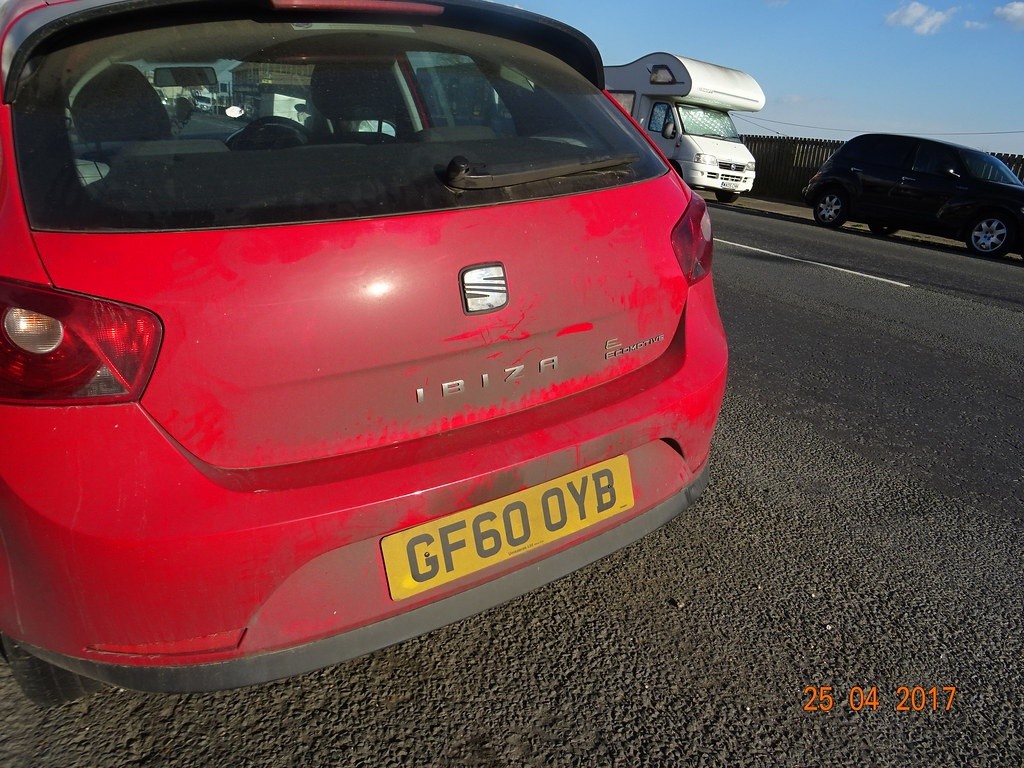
left=800, top=133, right=1024, bottom=261
left=1, top=0, right=728, bottom=714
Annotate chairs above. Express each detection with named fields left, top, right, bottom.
left=56, top=58, right=498, bottom=161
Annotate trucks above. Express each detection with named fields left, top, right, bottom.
left=602, top=51, right=767, bottom=203
